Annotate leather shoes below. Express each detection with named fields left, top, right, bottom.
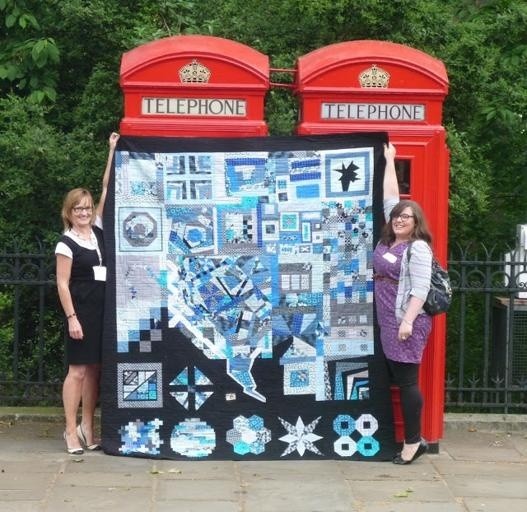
left=393, top=439, right=429, bottom=464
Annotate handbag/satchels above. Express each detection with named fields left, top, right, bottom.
left=423, top=257, right=453, bottom=316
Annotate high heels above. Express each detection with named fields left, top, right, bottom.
left=63, top=424, right=102, bottom=454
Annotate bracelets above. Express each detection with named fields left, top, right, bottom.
left=403, top=318, right=413, bottom=327
left=65, top=312, right=77, bottom=320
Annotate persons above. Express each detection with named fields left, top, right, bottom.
left=52, top=131, right=122, bottom=455
left=371, top=139, right=436, bottom=468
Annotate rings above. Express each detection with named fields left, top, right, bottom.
left=401, top=337, right=406, bottom=340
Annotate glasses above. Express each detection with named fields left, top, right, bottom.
left=399, top=214, right=414, bottom=222
left=72, top=207, right=93, bottom=213
